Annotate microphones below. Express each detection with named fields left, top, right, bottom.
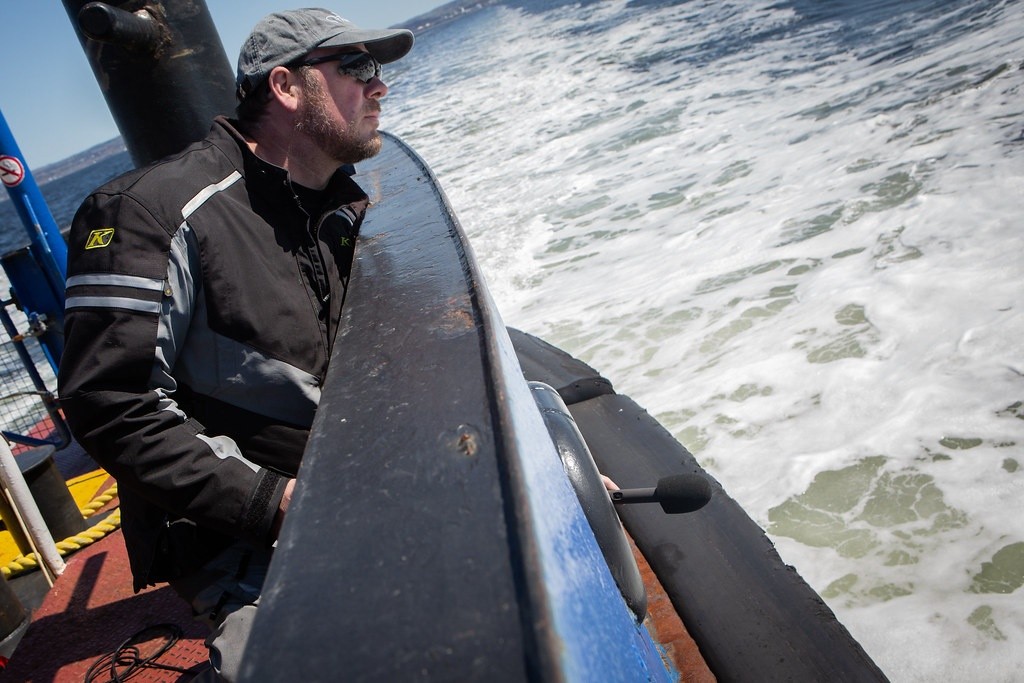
left=606, top=474, right=712, bottom=514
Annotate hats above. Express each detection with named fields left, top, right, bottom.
left=237, top=8, right=415, bottom=100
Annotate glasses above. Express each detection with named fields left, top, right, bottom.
left=290, top=50, right=383, bottom=84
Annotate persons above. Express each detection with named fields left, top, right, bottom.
left=57, top=8, right=415, bottom=683
